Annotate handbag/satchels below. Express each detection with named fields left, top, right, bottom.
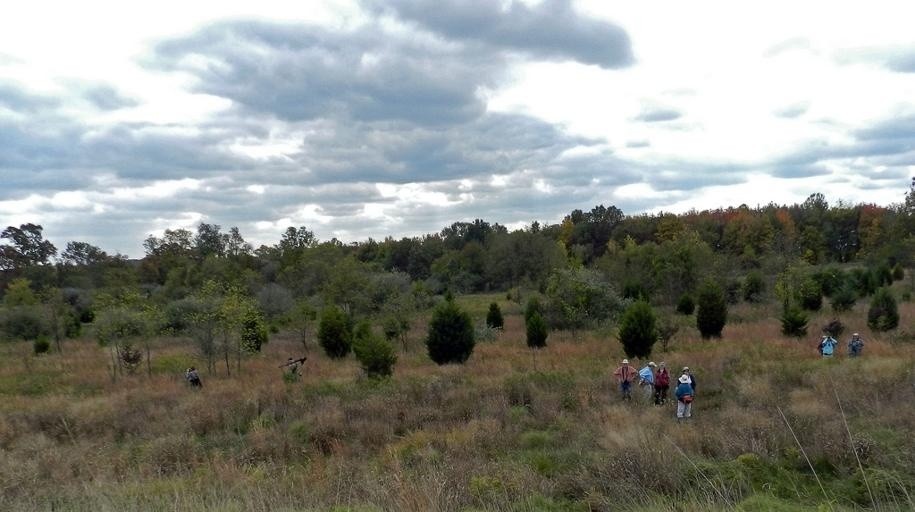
left=683, top=394, right=692, bottom=404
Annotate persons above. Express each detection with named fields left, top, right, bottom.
left=675, top=374, right=695, bottom=417
left=614, top=358, right=639, bottom=400
left=822, top=332, right=839, bottom=356
left=185, top=366, right=201, bottom=386
left=848, top=332, right=865, bottom=355
left=286, top=357, right=299, bottom=375
left=654, top=361, right=671, bottom=406
left=639, top=360, right=658, bottom=402
left=677, top=367, right=696, bottom=396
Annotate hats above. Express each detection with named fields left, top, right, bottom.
left=679, top=374, right=691, bottom=384
left=648, top=362, right=657, bottom=368
left=621, top=359, right=629, bottom=364
left=681, top=366, right=691, bottom=373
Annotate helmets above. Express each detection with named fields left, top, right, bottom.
left=853, top=333, right=859, bottom=340
